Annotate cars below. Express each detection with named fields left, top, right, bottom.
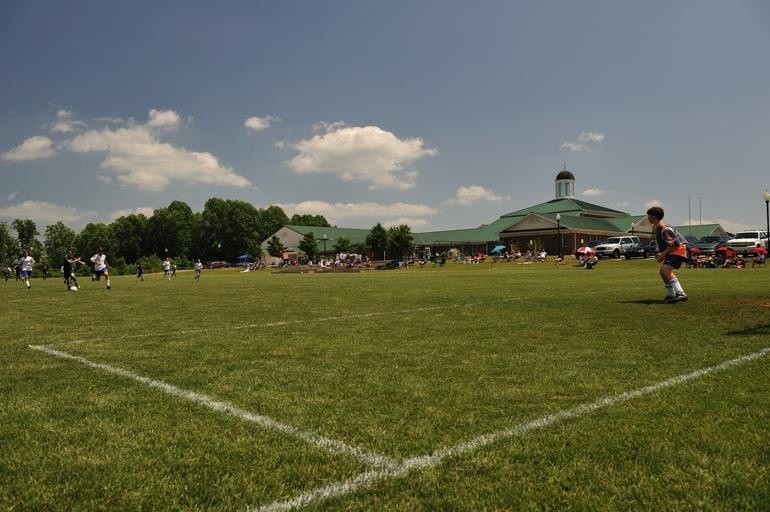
left=574, top=229, right=770, bottom=261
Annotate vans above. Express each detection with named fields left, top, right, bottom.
left=207, top=260, right=233, bottom=269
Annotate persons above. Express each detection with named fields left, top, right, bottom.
left=136, top=264, right=144, bottom=281
left=214, top=257, right=263, bottom=271
left=279, top=249, right=598, bottom=270
left=63, top=248, right=84, bottom=289
left=4, top=263, right=49, bottom=281
left=90, top=247, right=112, bottom=288
left=19, top=250, right=35, bottom=288
left=689, top=244, right=766, bottom=268
left=646, top=206, right=690, bottom=304
left=163, top=257, right=177, bottom=278
left=194, top=258, right=203, bottom=279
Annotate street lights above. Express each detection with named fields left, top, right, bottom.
left=762, top=187, right=770, bottom=260
left=529, top=239, right=534, bottom=253
left=322, top=234, right=328, bottom=259
left=630, top=222, right=635, bottom=236
left=555, top=213, right=563, bottom=259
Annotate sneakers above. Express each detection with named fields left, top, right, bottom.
left=661, top=291, right=687, bottom=302
